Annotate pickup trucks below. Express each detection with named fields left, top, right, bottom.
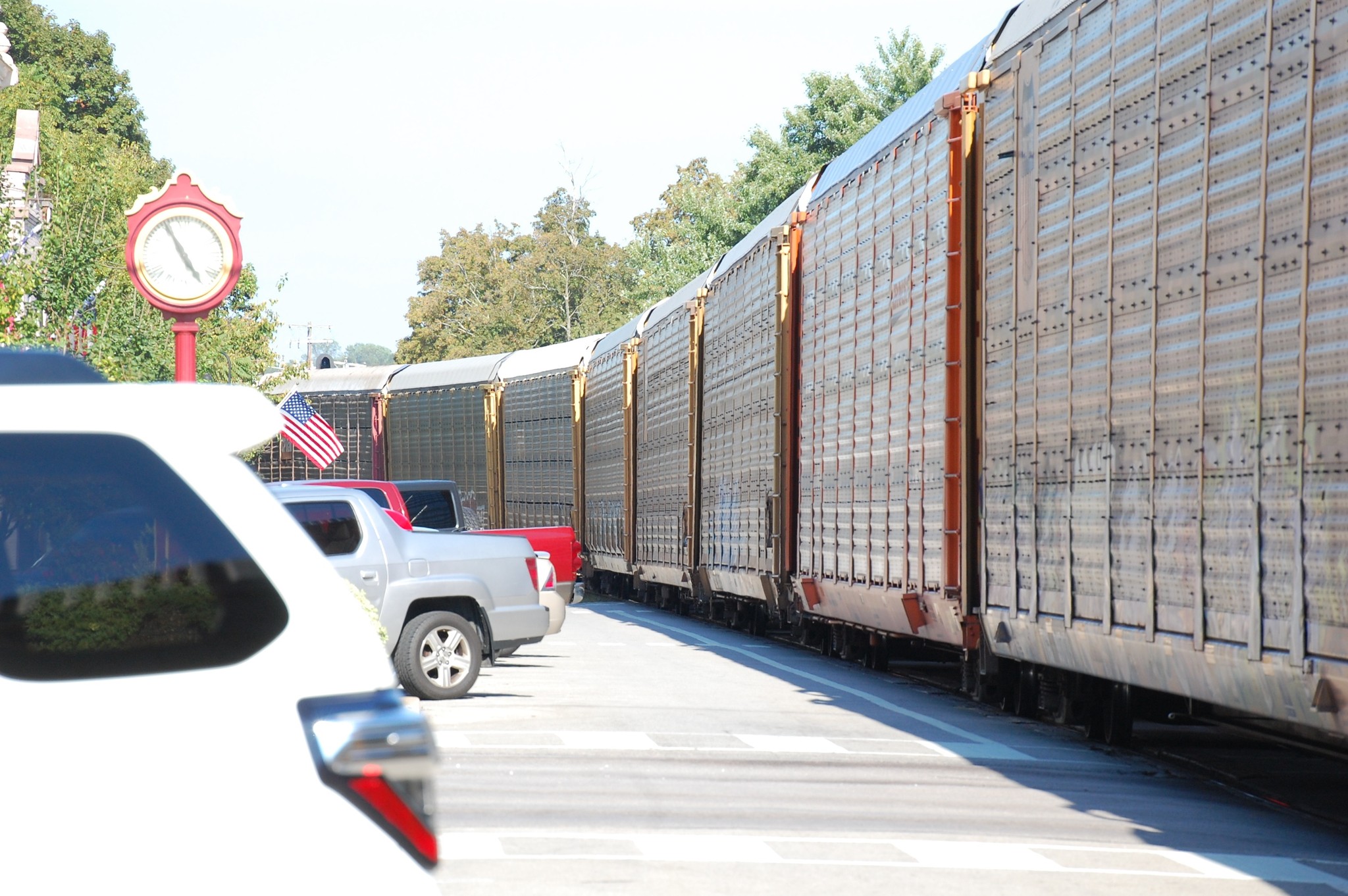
left=268, top=479, right=586, bottom=613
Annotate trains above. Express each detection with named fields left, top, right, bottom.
left=259, top=0, right=1347, bottom=751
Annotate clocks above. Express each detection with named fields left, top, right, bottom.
left=123, top=171, right=244, bottom=382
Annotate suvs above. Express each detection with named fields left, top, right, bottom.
left=390, top=479, right=469, bottom=534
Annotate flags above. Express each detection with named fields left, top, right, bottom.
left=279, top=391, right=344, bottom=472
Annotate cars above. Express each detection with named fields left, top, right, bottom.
left=1, top=376, right=439, bottom=891
left=263, top=484, right=551, bottom=701
